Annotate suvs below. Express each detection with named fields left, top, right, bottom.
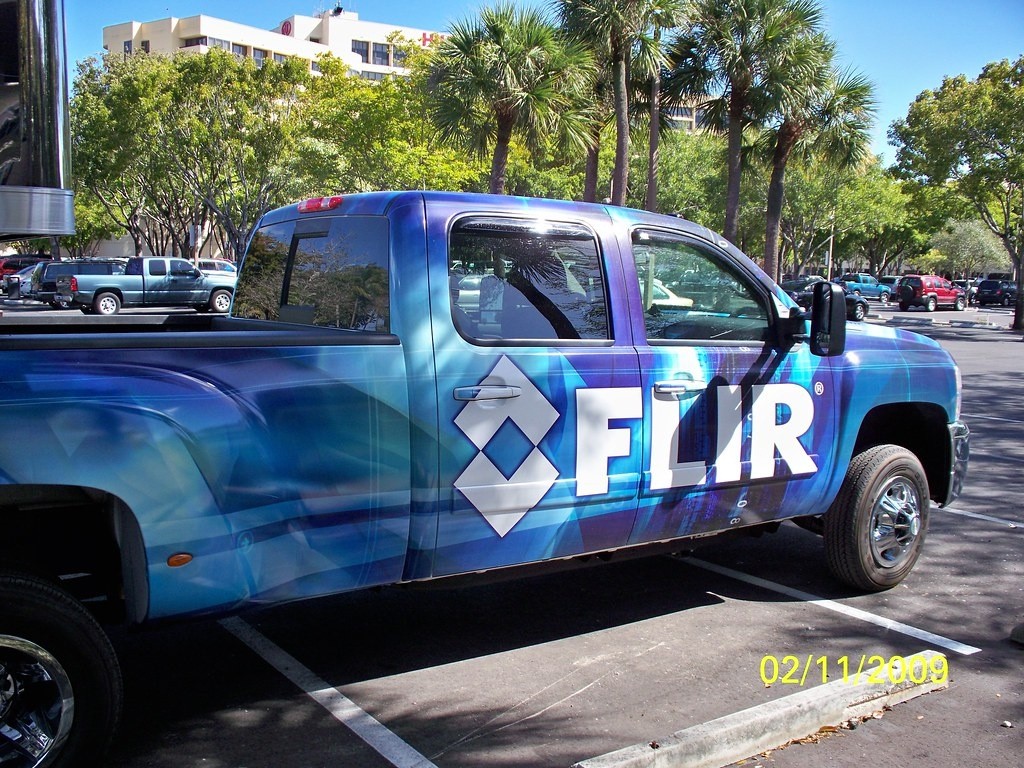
left=951, top=277, right=1018, bottom=307
left=880, top=274, right=965, bottom=312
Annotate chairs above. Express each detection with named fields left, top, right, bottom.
left=451, top=272, right=478, bottom=337
left=496, top=251, right=607, bottom=339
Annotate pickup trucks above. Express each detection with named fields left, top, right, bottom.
left=1, top=253, right=238, bottom=315
left=777, top=273, right=893, bottom=321
left=1, top=191, right=968, bottom=767
